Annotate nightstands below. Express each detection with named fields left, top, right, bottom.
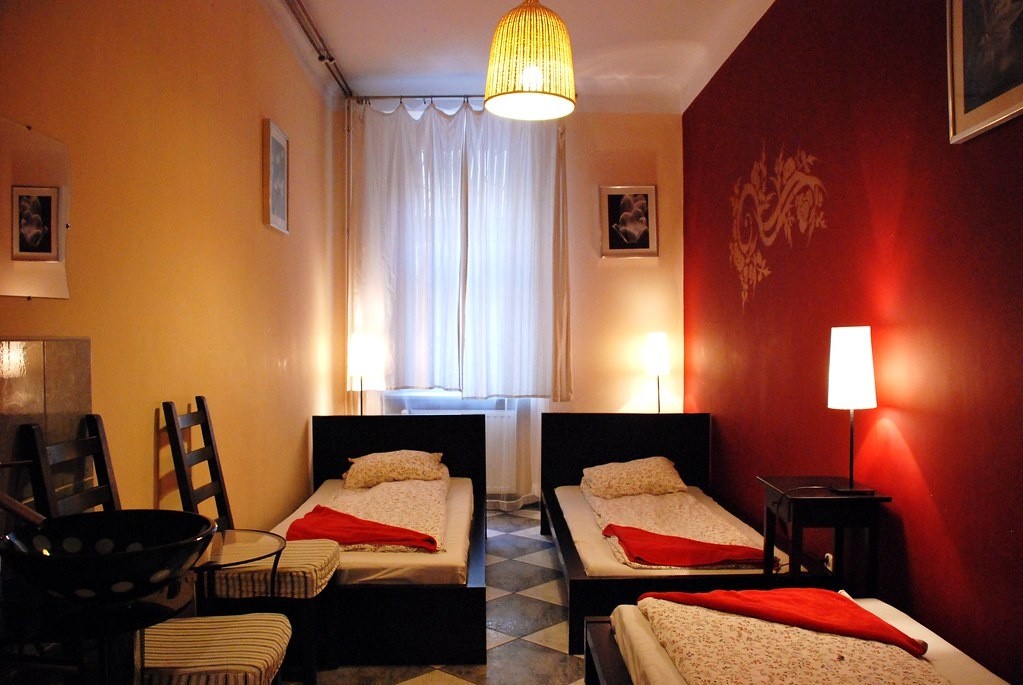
left=758, top=476, right=895, bottom=590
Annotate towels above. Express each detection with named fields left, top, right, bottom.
left=286, top=503, right=440, bottom=554
left=603, top=524, right=780, bottom=566
left=638, top=586, right=929, bottom=656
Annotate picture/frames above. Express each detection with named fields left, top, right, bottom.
left=597, top=182, right=660, bottom=258
left=946, top=1, right=1023, bottom=148
left=9, top=185, right=62, bottom=262
left=260, top=119, right=292, bottom=234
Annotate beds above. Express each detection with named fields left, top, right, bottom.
left=260, top=413, right=491, bottom=667
left=582, top=587, right=1008, bottom=685
left=539, top=412, right=813, bottom=655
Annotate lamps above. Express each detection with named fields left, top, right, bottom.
left=825, top=324, right=880, bottom=495
left=484, top=1, right=578, bottom=122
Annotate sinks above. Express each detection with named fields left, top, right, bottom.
left=0, top=510, right=219, bottom=623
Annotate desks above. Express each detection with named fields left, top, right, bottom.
left=180, top=530, right=286, bottom=619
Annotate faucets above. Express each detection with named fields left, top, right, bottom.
left=0, top=461, right=48, bottom=530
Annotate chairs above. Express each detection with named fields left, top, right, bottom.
left=18, top=412, right=292, bottom=685
left=163, top=396, right=340, bottom=685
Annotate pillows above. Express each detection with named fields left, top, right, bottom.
left=343, top=449, right=442, bottom=490
left=583, top=455, right=688, bottom=499
